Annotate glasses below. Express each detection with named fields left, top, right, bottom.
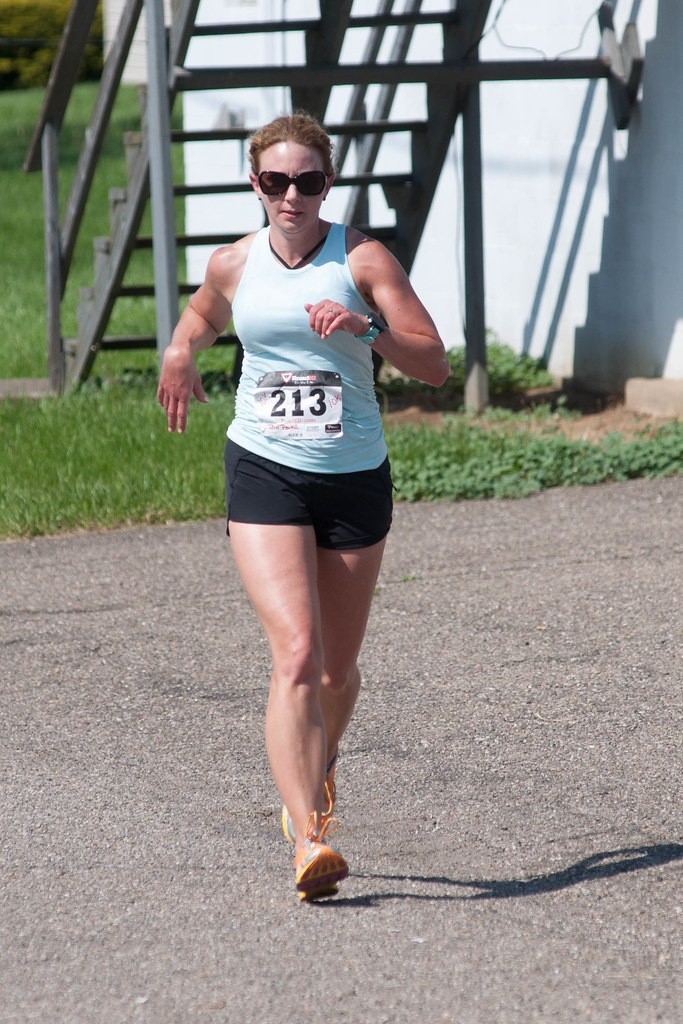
left=258, top=170, right=327, bottom=196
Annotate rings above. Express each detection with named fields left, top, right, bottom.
left=329, top=307, right=339, bottom=317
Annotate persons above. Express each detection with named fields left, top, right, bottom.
left=156, top=112, right=450, bottom=902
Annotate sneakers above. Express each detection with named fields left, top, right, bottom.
left=281, top=753, right=349, bottom=903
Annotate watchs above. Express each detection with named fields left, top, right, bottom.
left=354, top=311, right=386, bottom=345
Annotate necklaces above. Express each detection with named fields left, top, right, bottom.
left=270, top=236, right=326, bottom=270
